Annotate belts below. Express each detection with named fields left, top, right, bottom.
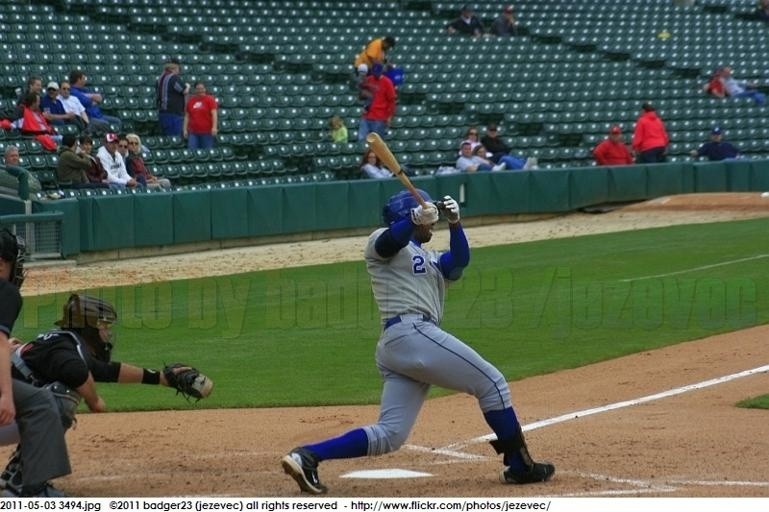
left=383, top=314, right=428, bottom=331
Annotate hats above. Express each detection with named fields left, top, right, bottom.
left=105, top=134, right=119, bottom=143
left=47, top=82, right=58, bottom=90
left=165, top=55, right=179, bottom=64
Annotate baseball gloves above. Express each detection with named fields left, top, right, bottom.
left=164, top=363, right=214, bottom=397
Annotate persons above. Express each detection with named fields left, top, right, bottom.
left=281, top=189, right=556, bottom=494
left=1, top=227, right=28, bottom=427
left=0, top=293, right=194, bottom=497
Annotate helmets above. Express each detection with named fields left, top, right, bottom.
left=0, top=226, right=28, bottom=286
left=54, top=293, right=116, bottom=362
left=384, top=190, right=437, bottom=224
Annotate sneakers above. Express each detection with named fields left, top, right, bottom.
left=499, top=463, right=555, bottom=483
left=0, top=477, right=65, bottom=497
left=280, top=446, right=327, bottom=493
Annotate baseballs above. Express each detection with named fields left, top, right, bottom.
left=761, top=191, right=768, bottom=199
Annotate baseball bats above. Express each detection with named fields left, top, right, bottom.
left=366, top=132, right=429, bottom=212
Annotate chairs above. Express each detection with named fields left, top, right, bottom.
left=0, top=0, right=769, bottom=202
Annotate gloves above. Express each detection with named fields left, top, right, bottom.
left=437, top=196, right=461, bottom=223
left=411, top=202, right=439, bottom=226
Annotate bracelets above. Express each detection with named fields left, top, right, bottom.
left=142, top=368, right=159, bottom=385
